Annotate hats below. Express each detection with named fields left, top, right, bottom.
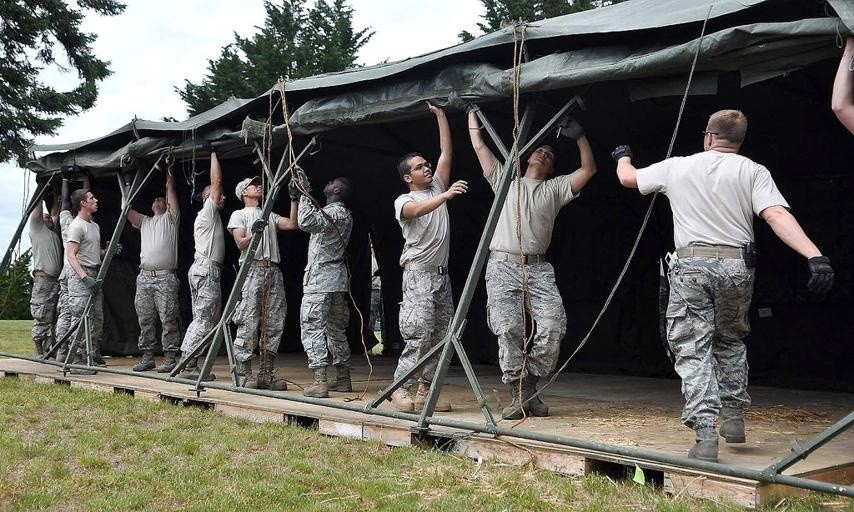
left=234, top=175, right=262, bottom=202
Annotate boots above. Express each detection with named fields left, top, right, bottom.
left=235, top=360, right=257, bottom=389
left=256, top=355, right=288, bottom=390
left=302, top=367, right=329, bottom=399
left=152, top=350, right=177, bottom=373
left=70, top=341, right=97, bottom=375
left=502, top=378, right=529, bottom=420
left=687, top=420, right=719, bottom=464
left=415, top=383, right=451, bottom=412
left=718, top=410, right=745, bottom=443
left=33, top=339, right=44, bottom=361
left=132, top=348, right=155, bottom=372
left=526, top=375, right=549, bottom=417
left=327, top=366, right=352, bottom=393
left=176, top=356, right=216, bottom=381
left=390, top=387, right=414, bottom=413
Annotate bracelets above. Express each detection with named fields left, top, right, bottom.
left=62, top=178, right=68, bottom=181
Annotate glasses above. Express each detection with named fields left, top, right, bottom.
left=702, top=131, right=719, bottom=136
left=408, top=162, right=432, bottom=175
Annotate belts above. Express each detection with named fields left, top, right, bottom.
left=404, top=263, right=449, bottom=275
left=140, top=269, right=173, bottom=276
left=674, top=246, right=747, bottom=259
left=489, top=251, right=545, bottom=266
left=34, top=277, right=57, bottom=284
left=193, top=258, right=221, bottom=268
left=250, top=260, right=274, bottom=267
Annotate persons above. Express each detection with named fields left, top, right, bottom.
left=55, top=166, right=90, bottom=363
left=467, top=108, right=598, bottom=421
left=385, top=101, right=469, bottom=414
left=612, top=108, right=836, bottom=463
left=369, top=248, right=384, bottom=356
left=830, top=37, right=854, bottom=137
left=121, top=160, right=181, bottom=374
left=178, top=147, right=227, bottom=382
left=29, top=195, right=63, bottom=363
left=68, top=189, right=122, bottom=375
left=228, top=175, right=298, bottom=391
left=294, top=163, right=354, bottom=399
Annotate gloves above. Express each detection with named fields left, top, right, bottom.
left=82, top=275, right=102, bottom=297
left=555, top=116, right=585, bottom=140
left=447, top=91, right=472, bottom=116
left=104, top=242, right=123, bottom=256
left=287, top=177, right=300, bottom=201
left=807, top=256, right=834, bottom=295
left=611, top=144, right=634, bottom=163
left=294, top=165, right=313, bottom=194
left=202, top=140, right=218, bottom=154
left=60, top=165, right=69, bottom=181
left=251, top=219, right=269, bottom=236
